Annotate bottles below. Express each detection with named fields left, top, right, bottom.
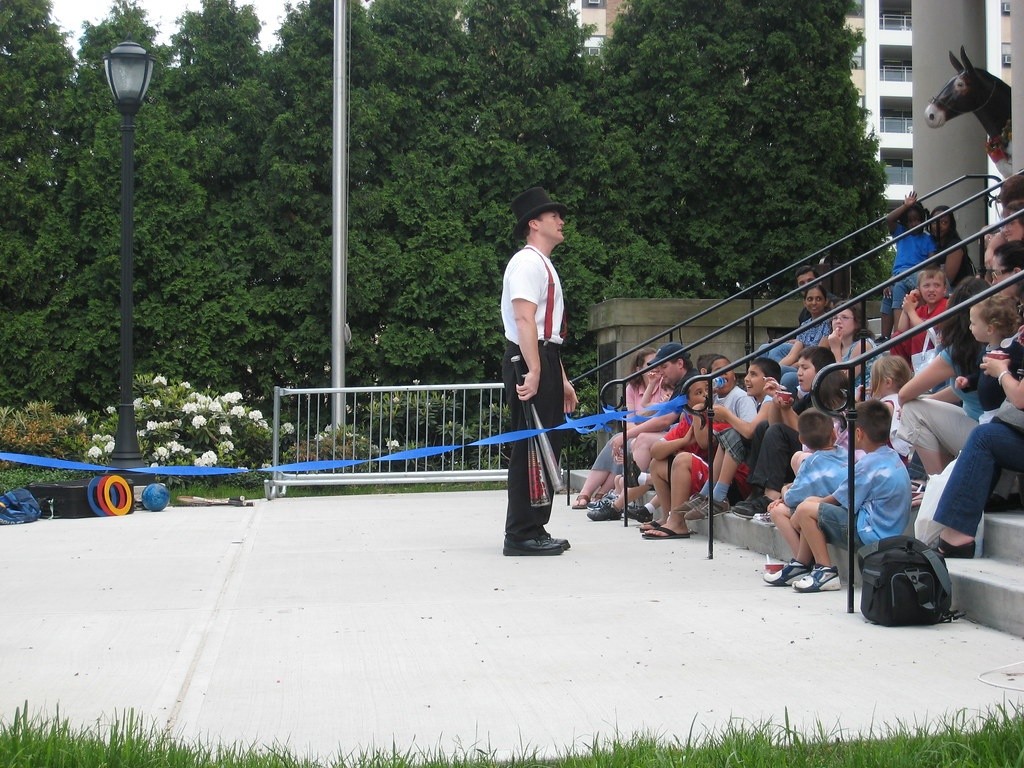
left=708, top=377, right=728, bottom=388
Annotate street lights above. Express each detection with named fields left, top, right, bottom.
left=100, top=42, right=156, bottom=483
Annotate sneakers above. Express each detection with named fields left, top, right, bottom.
left=685, top=495, right=731, bottom=520
left=672, top=492, right=707, bottom=512
left=763, top=558, right=811, bottom=586
left=731, top=487, right=767, bottom=519
left=628, top=505, right=653, bottom=523
left=587, top=502, right=624, bottom=521
left=792, top=563, right=840, bottom=591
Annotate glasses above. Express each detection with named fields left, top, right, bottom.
left=1006, top=220, right=1019, bottom=227
left=991, top=269, right=1013, bottom=282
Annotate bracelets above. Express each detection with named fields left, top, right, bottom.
left=998, top=371, right=1012, bottom=386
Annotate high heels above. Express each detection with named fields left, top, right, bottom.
left=924, top=535, right=976, bottom=559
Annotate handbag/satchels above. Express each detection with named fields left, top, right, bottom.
left=913, top=450, right=986, bottom=559
left=0, top=488, right=41, bottom=525
left=910, top=327, right=950, bottom=395
left=856, top=535, right=967, bottom=627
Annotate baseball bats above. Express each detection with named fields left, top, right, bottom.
left=511, top=354, right=564, bottom=507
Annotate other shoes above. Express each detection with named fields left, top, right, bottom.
left=587, top=488, right=620, bottom=511
left=873, top=336, right=889, bottom=345
left=752, top=512, right=775, bottom=527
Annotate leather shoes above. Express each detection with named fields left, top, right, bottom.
left=503, top=532, right=571, bottom=556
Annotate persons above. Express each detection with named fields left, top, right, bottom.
left=572, top=175, right=1024, bottom=593
left=501, top=187, right=579, bottom=556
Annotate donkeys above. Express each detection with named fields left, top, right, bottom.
left=924, top=46, right=1011, bottom=178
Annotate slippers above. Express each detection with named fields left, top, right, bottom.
left=572, top=493, right=604, bottom=509
left=640, top=521, right=690, bottom=539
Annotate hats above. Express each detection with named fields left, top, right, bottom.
left=511, top=187, right=567, bottom=241
left=646, top=343, right=692, bottom=366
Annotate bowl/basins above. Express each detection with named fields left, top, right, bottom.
left=765, top=564, right=784, bottom=574
left=775, top=390, right=792, bottom=403
left=986, top=352, right=1010, bottom=362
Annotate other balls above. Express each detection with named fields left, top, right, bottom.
left=142, top=483, right=170, bottom=511
